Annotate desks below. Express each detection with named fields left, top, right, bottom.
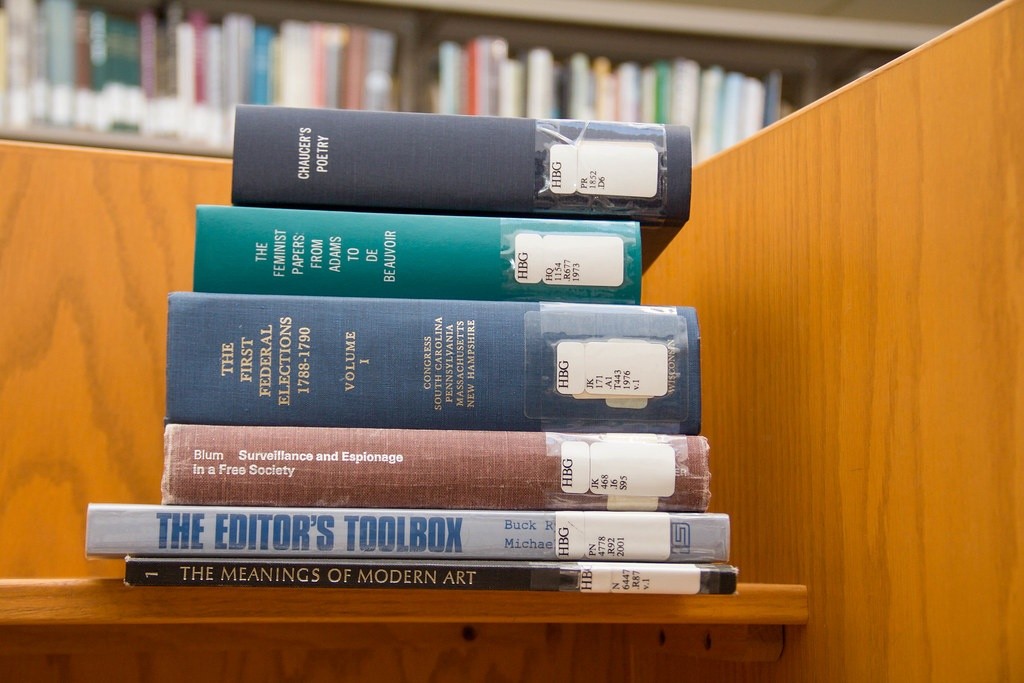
left=0, top=567, right=811, bottom=628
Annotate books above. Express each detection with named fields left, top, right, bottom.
left=0, top=0, right=804, bottom=190
left=82, top=102, right=750, bottom=596
